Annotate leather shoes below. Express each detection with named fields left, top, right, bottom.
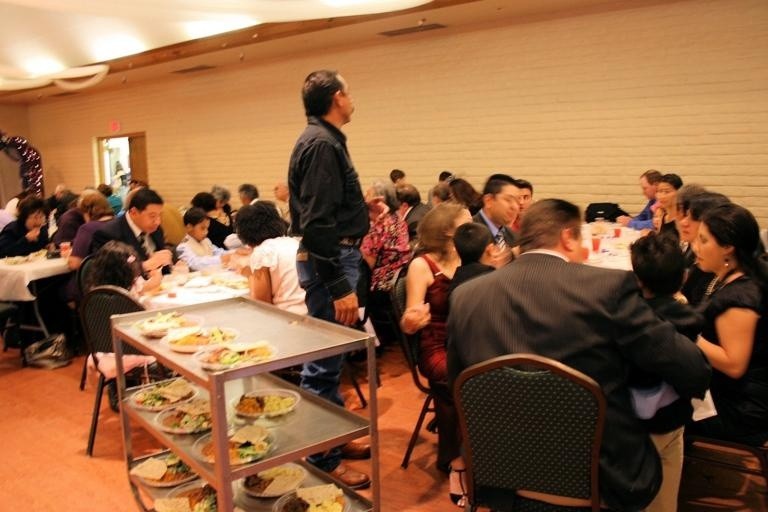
left=328, top=460, right=371, bottom=489
left=340, top=441, right=371, bottom=459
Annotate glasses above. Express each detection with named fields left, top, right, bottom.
left=33, top=213, right=45, bottom=221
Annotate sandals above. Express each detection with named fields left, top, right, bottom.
left=449, top=463, right=469, bottom=508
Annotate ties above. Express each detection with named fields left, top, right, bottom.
left=497, top=231, right=512, bottom=265
left=140, top=232, right=148, bottom=256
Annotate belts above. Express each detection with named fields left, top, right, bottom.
left=338, top=237, right=361, bottom=246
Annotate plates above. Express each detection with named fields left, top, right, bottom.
left=134, top=268, right=249, bottom=302
left=237, top=461, right=307, bottom=501
left=136, top=314, right=274, bottom=372
left=188, top=424, right=275, bottom=465
left=269, top=488, right=351, bottom=512
left=126, top=381, right=233, bottom=511
left=234, top=388, right=302, bottom=419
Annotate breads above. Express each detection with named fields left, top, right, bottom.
left=176, top=398, right=211, bottom=416
left=263, top=477, right=302, bottom=497
left=160, top=378, right=191, bottom=402
left=155, top=497, right=191, bottom=512
left=228, top=426, right=269, bottom=444
left=297, top=484, right=343, bottom=506
left=130, top=457, right=168, bottom=481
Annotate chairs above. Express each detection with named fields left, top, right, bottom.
left=76, top=255, right=97, bottom=389
left=389, top=264, right=462, bottom=468
left=83, top=285, right=157, bottom=457
left=585, top=203, right=632, bottom=223
left=682, top=433, right=767, bottom=511
left=452, top=354, right=609, bottom=512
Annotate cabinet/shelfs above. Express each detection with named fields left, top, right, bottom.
left=112, top=295, right=383, bottom=511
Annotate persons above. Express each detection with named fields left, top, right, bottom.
left=447, top=199, right=710, bottom=512
left=364, top=172, right=533, bottom=507
left=179, top=182, right=308, bottom=375
left=617, top=169, right=768, bottom=448
left=285, top=68, right=372, bottom=493
left=0, top=179, right=173, bottom=411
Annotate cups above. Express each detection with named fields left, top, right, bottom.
left=580, top=218, right=622, bottom=261
left=59, top=242, right=71, bottom=256
